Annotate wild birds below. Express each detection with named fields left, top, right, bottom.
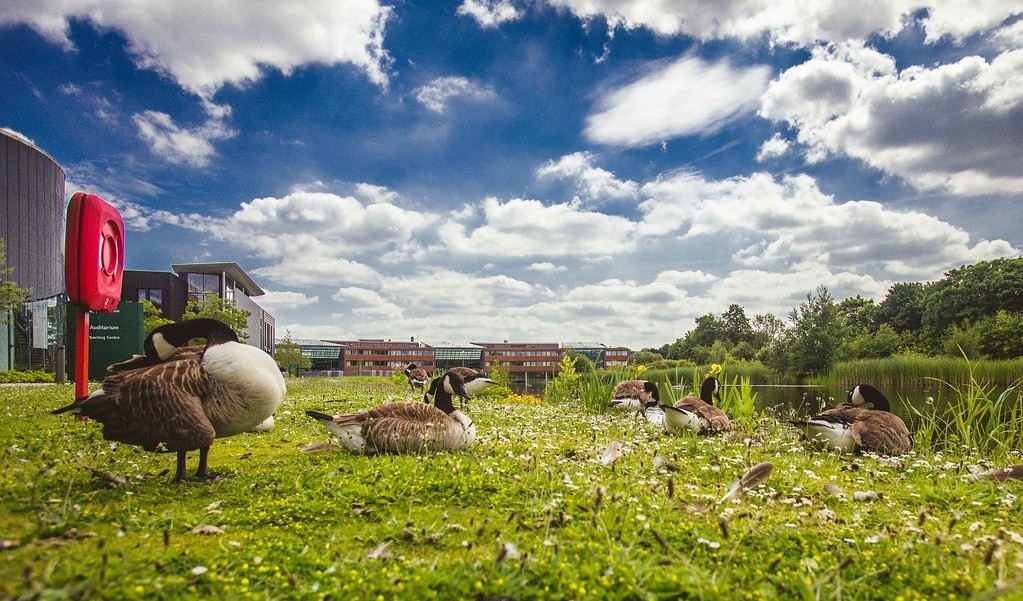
left=305, top=371, right=477, bottom=455
left=49, top=318, right=286, bottom=486
left=659, top=376, right=730, bottom=437
left=787, top=383, right=913, bottom=454
left=608, top=380, right=659, bottom=420
left=424, top=367, right=499, bottom=411
left=404, top=364, right=428, bottom=395
